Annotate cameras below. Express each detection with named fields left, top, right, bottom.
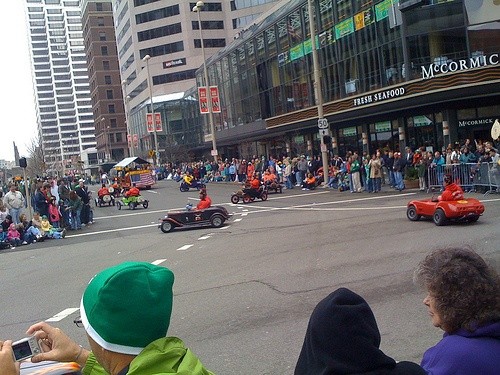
left=11, top=336, right=41, bottom=363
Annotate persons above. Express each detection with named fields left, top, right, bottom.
left=0, top=262, right=214, bottom=375
left=34, top=184, right=49, bottom=219
left=294, top=287, right=429, bottom=375
left=76, top=182, right=93, bottom=227
left=413, top=245, right=500, bottom=375
left=350, top=157, right=362, bottom=193
left=367, top=154, right=382, bottom=193
left=437, top=175, right=463, bottom=201
left=458, top=148, right=476, bottom=192
left=0, top=138, right=500, bottom=250
left=48, top=199, right=61, bottom=228
left=430, top=151, right=445, bottom=192
left=65, top=191, right=83, bottom=230
left=2, top=186, right=25, bottom=224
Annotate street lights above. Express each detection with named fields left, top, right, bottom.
left=191, top=1, right=218, bottom=164
left=142, top=55, right=158, bottom=164
left=121, top=79, right=135, bottom=156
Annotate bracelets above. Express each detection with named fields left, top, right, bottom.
left=73, top=345, right=83, bottom=361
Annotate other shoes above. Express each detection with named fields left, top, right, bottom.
left=302, top=188, right=305, bottom=191
left=92, top=221, right=96, bottom=224
left=307, top=188, right=310, bottom=191
left=88, top=221, right=92, bottom=225
left=62, top=228, right=67, bottom=238
left=37, top=236, right=44, bottom=242
left=81, top=223, right=86, bottom=227
left=22, top=241, right=28, bottom=245
left=77, top=227, right=81, bottom=230
left=295, top=184, right=300, bottom=186
left=300, top=185, right=303, bottom=187
left=33, top=240, right=36, bottom=243
left=13, top=242, right=21, bottom=247
left=71, top=227, right=76, bottom=230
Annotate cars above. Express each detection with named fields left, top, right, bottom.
left=229, top=180, right=281, bottom=205
left=93, top=186, right=150, bottom=211
left=406, top=196, right=486, bottom=227
left=157, top=202, right=234, bottom=233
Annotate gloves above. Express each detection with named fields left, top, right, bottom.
left=52, top=214, right=56, bottom=219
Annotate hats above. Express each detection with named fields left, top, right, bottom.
left=79, top=179, right=85, bottom=183
left=443, top=174, right=452, bottom=184
left=80, top=261, right=175, bottom=355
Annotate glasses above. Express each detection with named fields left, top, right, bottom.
left=73, top=316, right=83, bottom=329
